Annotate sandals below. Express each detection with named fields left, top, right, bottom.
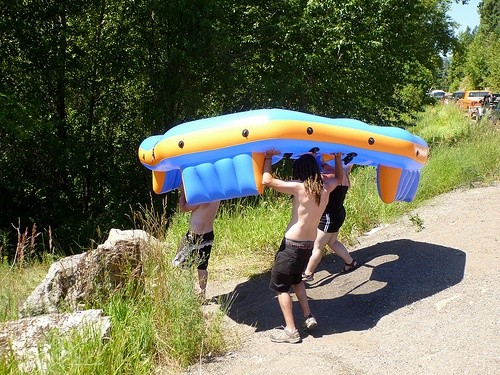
left=301, top=272, right=313, bottom=281
left=339, top=259, right=359, bottom=274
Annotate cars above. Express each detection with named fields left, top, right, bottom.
left=428, top=90, right=500, bottom=120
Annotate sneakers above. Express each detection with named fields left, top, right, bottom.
left=270, top=326, right=302, bottom=344
left=302, top=316, right=318, bottom=331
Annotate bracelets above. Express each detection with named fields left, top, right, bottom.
left=321, top=163, right=327, bottom=168
left=265, top=157, right=272, bottom=160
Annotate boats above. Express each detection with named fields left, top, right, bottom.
left=138, top=106, right=430, bottom=206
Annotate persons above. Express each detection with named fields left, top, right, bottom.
left=175, top=182, right=220, bottom=304
left=262, top=148, right=343, bottom=343
left=302, top=152, right=359, bottom=281
